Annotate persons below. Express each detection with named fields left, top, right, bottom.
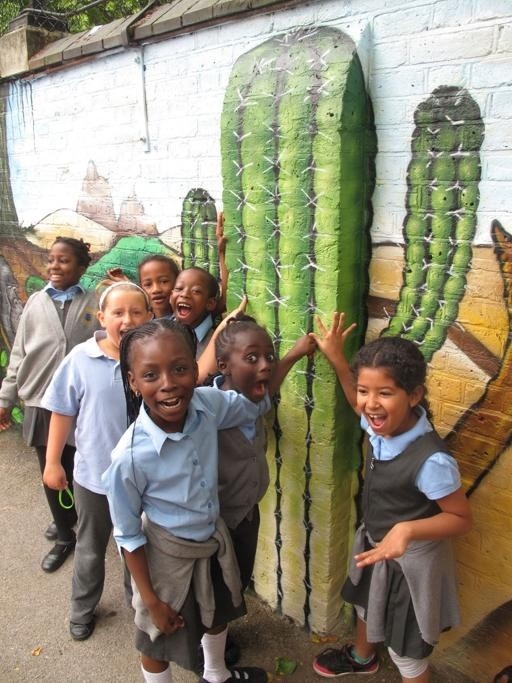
left=308, top=310, right=474, bottom=683
left=0, top=212, right=318, bottom=683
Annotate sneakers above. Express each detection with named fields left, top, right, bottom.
left=311, top=642, right=380, bottom=678
left=41, top=532, right=76, bottom=573
left=201, top=665, right=270, bottom=682
left=45, top=506, right=78, bottom=541
left=69, top=597, right=96, bottom=640
